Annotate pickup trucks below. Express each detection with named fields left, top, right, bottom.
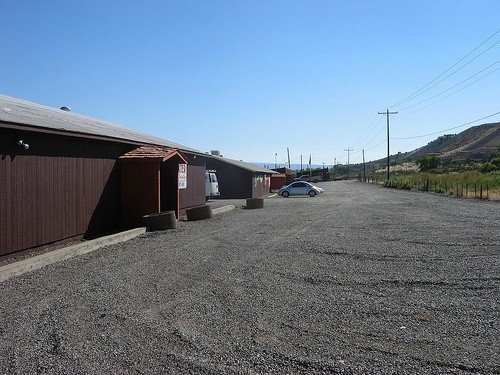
left=293, top=174, right=321, bottom=184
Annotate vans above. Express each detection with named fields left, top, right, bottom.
left=205, top=169, right=220, bottom=202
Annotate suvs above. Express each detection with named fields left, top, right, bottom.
left=335, top=174, right=341, bottom=180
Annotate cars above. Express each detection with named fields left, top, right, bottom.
left=278, top=180, right=320, bottom=198
left=281, top=183, right=324, bottom=193
